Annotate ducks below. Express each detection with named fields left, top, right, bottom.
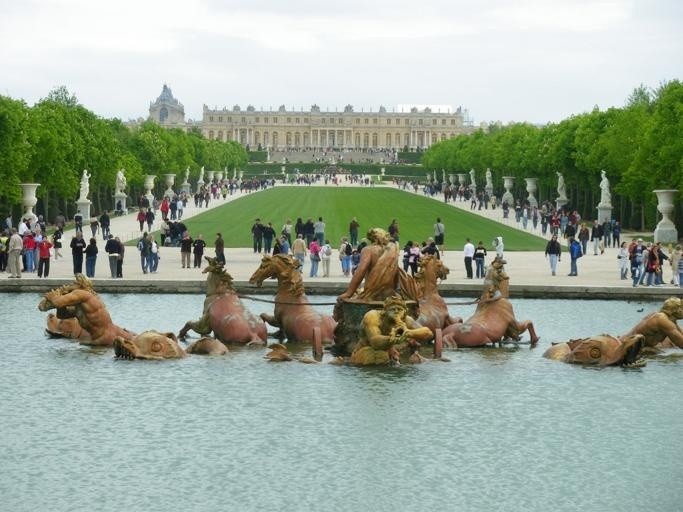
left=637, top=308, right=644, bottom=312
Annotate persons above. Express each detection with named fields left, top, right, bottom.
left=0, top=146, right=683, bottom=288
left=37, top=282, right=115, bottom=345
left=628, top=295, right=682, bottom=359
left=348, top=295, right=433, bottom=368
left=338, top=228, right=399, bottom=302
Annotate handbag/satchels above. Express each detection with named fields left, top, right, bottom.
left=617, top=255, right=621, bottom=259
left=313, top=252, right=321, bottom=261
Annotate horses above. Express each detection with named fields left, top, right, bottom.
left=178, top=256, right=268, bottom=344
left=440, top=254, right=538, bottom=349
left=413, top=254, right=464, bottom=337
left=38, top=273, right=178, bottom=348
left=249, top=252, right=337, bottom=344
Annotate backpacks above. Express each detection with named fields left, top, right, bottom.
left=325, top=245, right=331, bottom=255
left=138, top=241, right=144, bottom=250
left=344, top=243, right=351, bottom=255
